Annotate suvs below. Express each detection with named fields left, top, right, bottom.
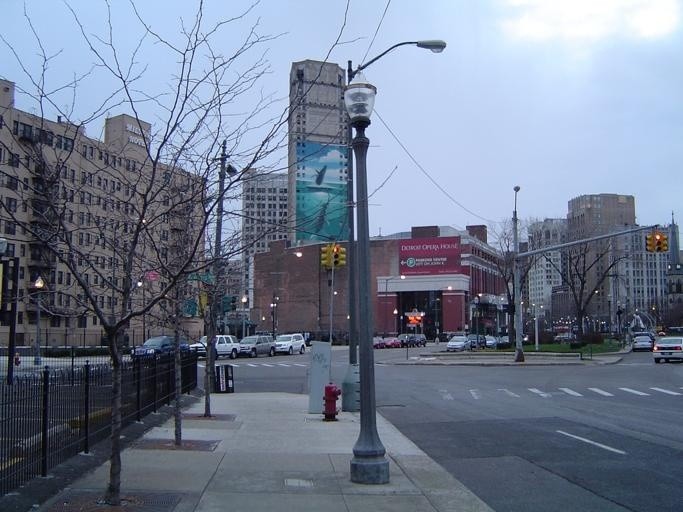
left=239, top=336, right=276, bottom=357
left=274, top=333, right=306, bottom=355
left=399, top=333, right=426, bottom=348
left=190, top=334, right=241, bottom=359
left=130, top=336, right=189, bottom=364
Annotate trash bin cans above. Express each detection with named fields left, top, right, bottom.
left=216, top=364, right=234, bottom=393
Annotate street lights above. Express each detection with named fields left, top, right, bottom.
left=271, top=294, right=279, bottom=338
left=0, top=237, right=8, bottom=261
left=430, top=298, right=439, bottom=337
left=607, top=293, right=612, bottom=337
left=343, top=39, right=450, bottom=413
left=616, top=296, right=629, bottom=350
left=434, top=285, right=452, bottom=345
left=275, top=251, right=302, bottom=329
left=399, top=315, right=403, bottom=334
left=203, top=137, right=238, bottom=393
left=241, top=295, right=248, bottom=338
left=384, top=274, right=405, bottom=336
left=468, top=293, right=543, bottom=333
left=392, top=308, right=398, bottom=335
left=511, top=185, right=525, bottom=362
left=551, top=306, right=658, bottom=331
left=136, top=274, right=145, bottom=345
left=33, top=275, right=46, bottom=366
left=347, top=62, right=390, bottom=484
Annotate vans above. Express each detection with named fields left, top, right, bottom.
left=633, top=332, right=655, bottom=350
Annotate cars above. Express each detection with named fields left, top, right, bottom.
left=657, top=331, right=665, bottom=336
left=652, top=337, right=682, bottom=364
left=631, top=336, right=652, bottom=351
left=485, top=336, right=496, bottom=349
left=469, top=334, right=485, bottom=349
left=496, top=325, right=578, bottom=345
left=383, top=337, right=399, bottom=348
left=446, top=336, right=470, bottom=352
left=372, top=337, right=384, bottom=348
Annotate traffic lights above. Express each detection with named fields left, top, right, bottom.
left=218, top=290, right=237, bottom=320
left=320, top=244, right=331, bottom=266
left=502, top=301, right=515, bottom=317
left=644, top=233, right=654, bottom=252
left=661, top=234, right=668, bottom=251
left=332, top=245, right=340, bottom=267
left=654, top=233, right=662, bottom=252
left=338, top=246, right=346, bottom=267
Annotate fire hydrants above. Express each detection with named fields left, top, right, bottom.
left=321, top=381, right=341, bottom=421
left=14, top=352, right=21, bottom=367
